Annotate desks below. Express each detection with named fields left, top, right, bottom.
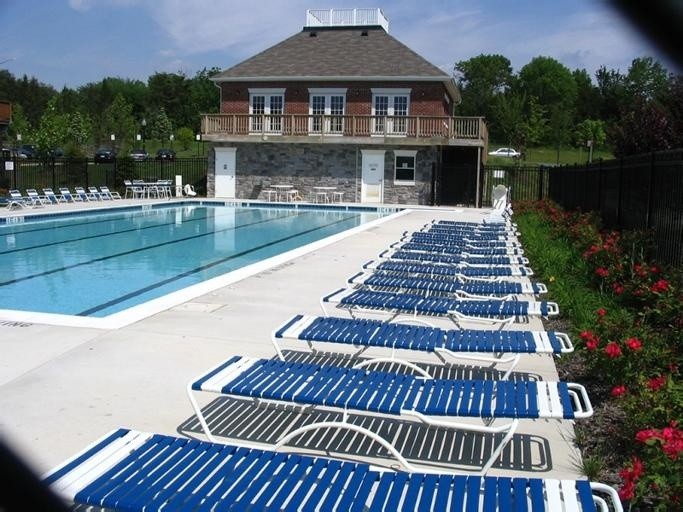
left=313, top=186, right=336, bottom=203
left=270, top=185, right=293, bottom=202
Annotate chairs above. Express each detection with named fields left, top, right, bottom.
left=39, top=426, right=621, bottom=512
left=185, top=354, right=596, bottom=481
left=268, top=310, right=577, bottom=383
left=0, top=180, right=172, bottom=210
left=320, top=219, right=559, bottom=330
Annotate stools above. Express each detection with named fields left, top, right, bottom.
left=262, top=190, right=343, bottom=204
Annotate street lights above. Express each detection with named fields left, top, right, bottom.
left=136, top=133, right=141, bottom=151
left=110, top=133, right=115, bottom=147
left=195, top=135, right=201, bottom=157
left=169, top=134, right=174, bottom=151
left=141, top=118, right=146, bottom=152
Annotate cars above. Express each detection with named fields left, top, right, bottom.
left=126, top=149, right=149, bottom=161
left=1, top=143, right=62, bottom=160
left=155, top=148, right=175, bottom=159
left=487, top=147, right=520, bottom=160
left=94, top=145, right=118, bottom=163
